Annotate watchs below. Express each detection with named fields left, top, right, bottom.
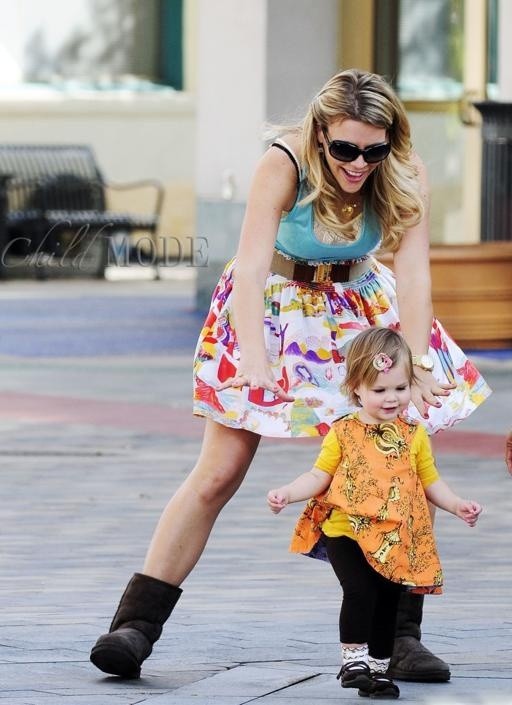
left=411, top=355, right=435, bottom=372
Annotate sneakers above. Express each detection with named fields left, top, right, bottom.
left=340, top=660, right=368, bottom=689
left=358, top=675, right=401, bottom=702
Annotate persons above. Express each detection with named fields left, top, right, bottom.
left=89, top=67, right=493, bottom=682
left=265, top=327, right=483, bottom=699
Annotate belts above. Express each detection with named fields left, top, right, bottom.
left=270, top=250, right=372, bottom=284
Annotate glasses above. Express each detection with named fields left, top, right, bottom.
left=318, top=121, right=393, bottom=162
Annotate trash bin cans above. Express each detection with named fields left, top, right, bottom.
left=472, top=101, right=512, bottom=243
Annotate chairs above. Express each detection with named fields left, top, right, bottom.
left=0, top=141, right=166, bottom=280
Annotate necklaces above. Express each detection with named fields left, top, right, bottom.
left=330, top=195, right=362, bottom=219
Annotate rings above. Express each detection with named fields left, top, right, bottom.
left=236, top=372, right=245, bottom=379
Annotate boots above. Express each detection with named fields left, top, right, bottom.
left=387, top=592, right=450, bottom=685
left=89, top=572, right=183, bottom=681
